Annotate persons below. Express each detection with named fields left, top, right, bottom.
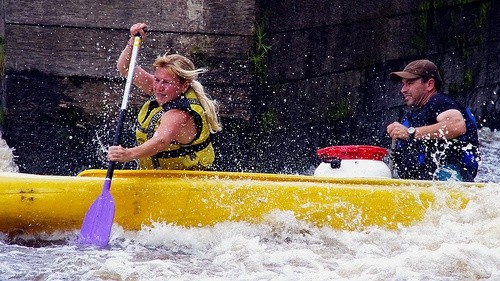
left=387, top=59, right=479, bottom=182
left=108, top=23, right=223, bottom=170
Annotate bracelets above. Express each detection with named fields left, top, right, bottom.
left=127, top=41, right=134, bottom=48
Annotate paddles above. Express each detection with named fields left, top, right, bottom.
left=387, top=103, right=408, bottom=178
left=75, top=24, right=147, bottom=251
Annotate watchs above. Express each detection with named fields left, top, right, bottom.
left=407, top=126, right=416, bottom=142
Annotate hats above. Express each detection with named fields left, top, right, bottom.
left=390, top=60, right=442, bottom=82
